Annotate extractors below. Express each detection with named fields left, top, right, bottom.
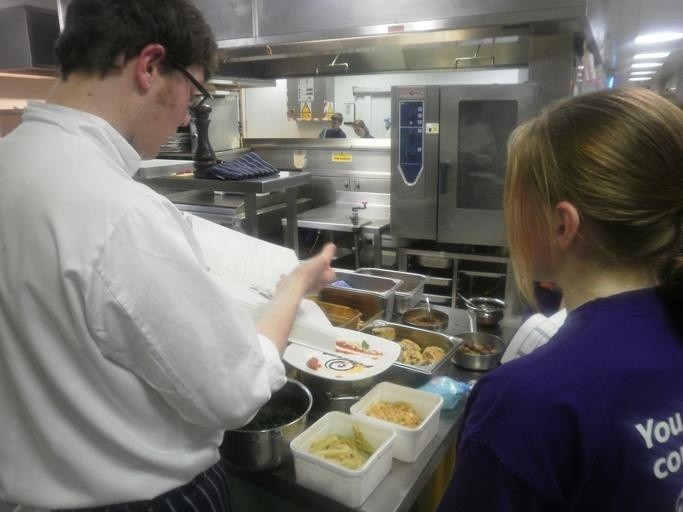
left=57, top=0, right=606, bottom=80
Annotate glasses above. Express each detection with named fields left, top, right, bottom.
left=164, top=52, right=212, bottom=110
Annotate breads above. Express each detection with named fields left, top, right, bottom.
left=372, top=325, right=444, bottom=369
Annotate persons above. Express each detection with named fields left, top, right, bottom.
left=318, top=112, right=345, bottom=138
left=433, top=86, right=682, bottom=512
left=351, top=119, right=374, bottom=138
left=0, top=0, right=336, bottom=512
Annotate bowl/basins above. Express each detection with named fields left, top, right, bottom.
left=220, top=375, right=312, bottom=474
left=451, top=331, right=506, bottom=371
left=401, top=307, right=448, bottom=331
left=465, top=296, right=506, bottom=326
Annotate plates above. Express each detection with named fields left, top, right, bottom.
left=280, top=325, right=400, bottom=381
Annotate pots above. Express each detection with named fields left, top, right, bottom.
left=309, top=376, right=378, bottom=419
